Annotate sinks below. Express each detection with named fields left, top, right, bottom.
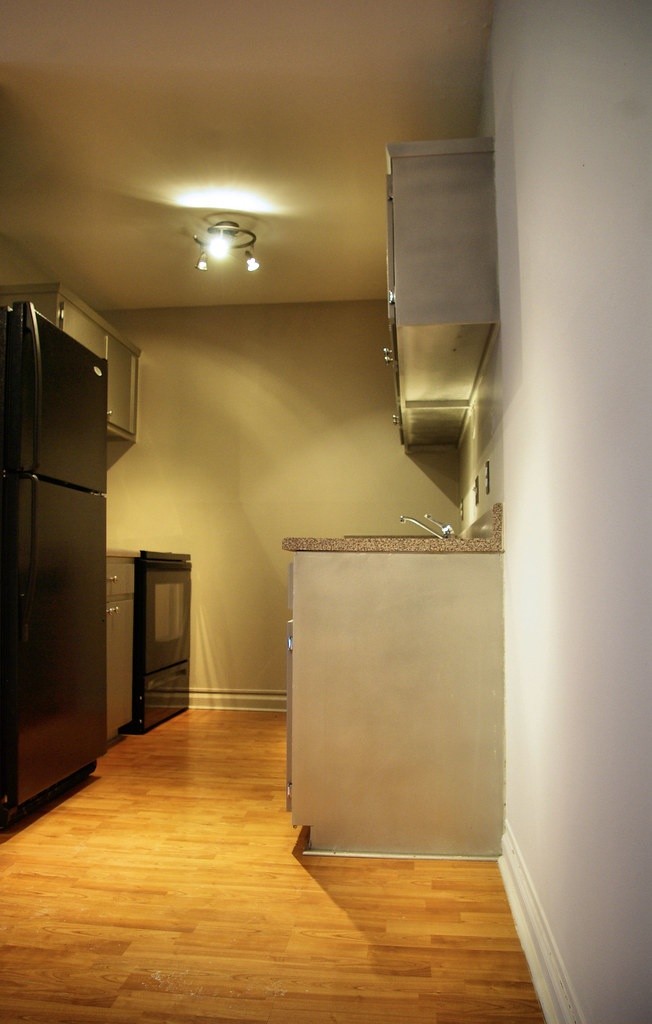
left=344, top=535, right=439, bottom=538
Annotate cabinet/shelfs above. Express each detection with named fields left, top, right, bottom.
left=383, top=136, right=499, bottom=326
left=106, top=547, right=141, bottom=747
left=384, top=322, right=495, bottom=455
left=282, top=536, right=505, bottom=861
left=0, top=283, right=142, bottom=444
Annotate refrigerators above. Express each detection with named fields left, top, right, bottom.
left=0, top=300, right=111, bottom=827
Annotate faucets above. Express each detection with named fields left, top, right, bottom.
left=400, top=513, right=456, bottom=539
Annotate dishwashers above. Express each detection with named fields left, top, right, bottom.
left=135, top=558, right=192, bottom=730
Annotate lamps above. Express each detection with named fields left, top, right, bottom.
left=191, top=220, right=260, bottom=272
